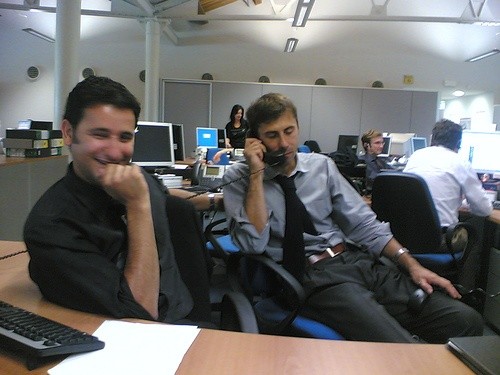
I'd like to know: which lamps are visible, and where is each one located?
[292,0,315,27]
[284,38,299,53]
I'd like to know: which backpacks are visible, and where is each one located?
[329,152,357,183]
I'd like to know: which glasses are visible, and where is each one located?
[371,141,386,145]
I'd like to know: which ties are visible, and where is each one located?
[272,171,322,312]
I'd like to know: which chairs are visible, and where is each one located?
[165,141,478,340]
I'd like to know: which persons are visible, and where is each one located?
[225,105,248,148]
[357,129,395,196]
[403,119,500,284]
[24,75,222,328]
[222,93,484,342]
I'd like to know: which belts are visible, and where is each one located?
[307,241,354,266]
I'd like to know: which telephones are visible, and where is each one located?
[230,148,247,163]
[192,162,227,191]
[245,129,286,167]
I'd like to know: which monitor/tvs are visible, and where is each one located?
[458,130,500,174]
[128,121,185,167]
[195,127,227,149]
[337,135,359,155]
[376,132,426,158]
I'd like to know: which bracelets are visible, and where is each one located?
[392,247,409,262]
[208,193,214,211]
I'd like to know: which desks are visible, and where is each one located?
[0,240,27,260]
[461,204,500,337]
[0,251,476,375]
[0,153,68,241]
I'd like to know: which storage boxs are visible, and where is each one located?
[0,121,64,158]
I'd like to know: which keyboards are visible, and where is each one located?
[0,301,105,371]
